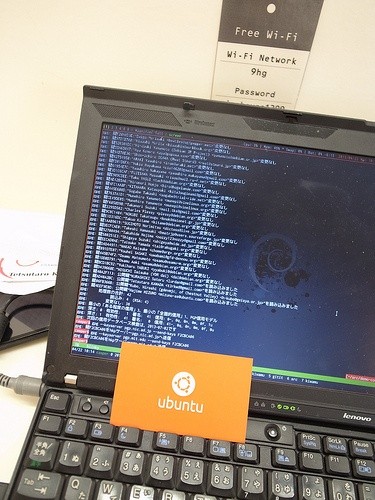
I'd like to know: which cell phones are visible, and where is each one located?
[1,285,56,352]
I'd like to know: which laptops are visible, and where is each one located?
[3,84,375,500]
